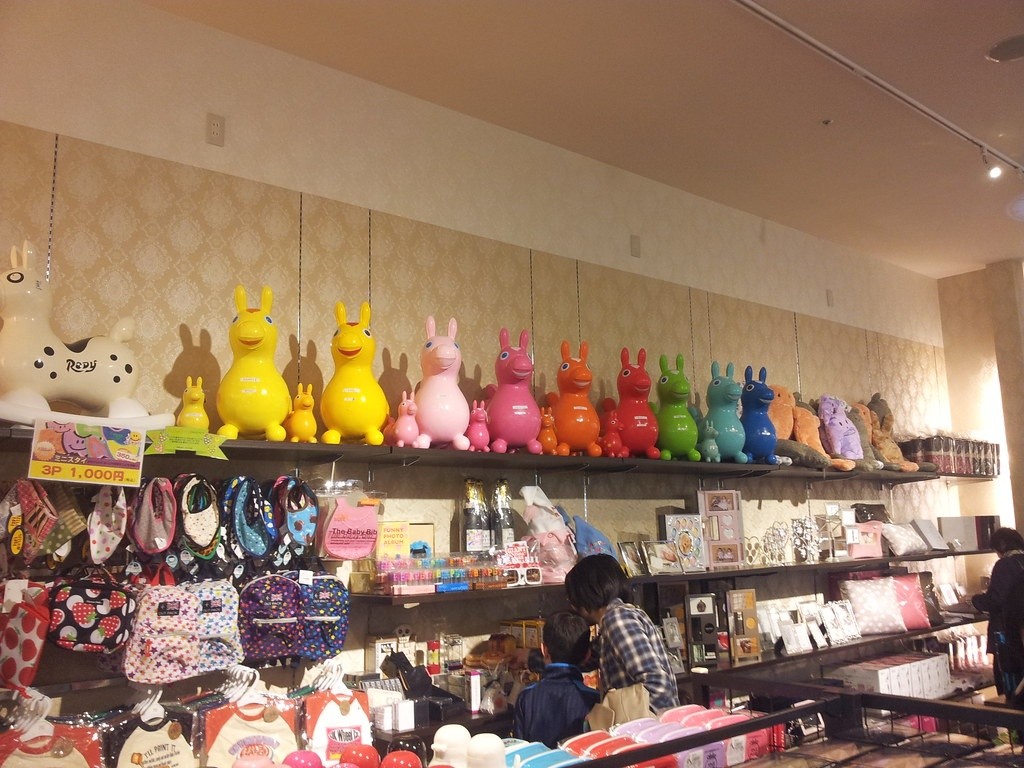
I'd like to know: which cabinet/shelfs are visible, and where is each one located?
[0,426,997,606]
[672,614,998,722]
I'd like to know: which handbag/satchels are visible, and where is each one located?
[0,579,50,691]
[49,552,352,686]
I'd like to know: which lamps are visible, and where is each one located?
[980,146,1003,179]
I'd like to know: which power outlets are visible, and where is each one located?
[205,112,226,148]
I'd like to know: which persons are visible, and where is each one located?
[504,611,601,750]
[972,527,1024,745]
[506,552,680,731]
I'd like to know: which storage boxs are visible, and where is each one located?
[365,636,399,680]
[910,519,950,552]
[821,651,984,732]
[937,516,966,552]
[829,566,909,600]
[464,671,480,714]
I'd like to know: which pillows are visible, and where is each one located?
[841,577,908,635]
[873,572,934,631]
[882,522,927,555]
[919,571,946,626]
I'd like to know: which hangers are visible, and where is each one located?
[309,658,353,699]
[6,686,53,741]
[127,677,166,721]
[218,664,267,708]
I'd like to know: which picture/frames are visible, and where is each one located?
[617,540,646,577]
[662,617,685,649]
[818,604,849,645]
[666,647,686,674]
[640,540,685,575]
[795,600,821,636]
[829,599,862,638]
